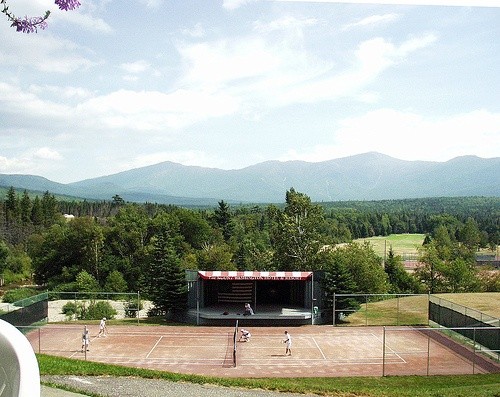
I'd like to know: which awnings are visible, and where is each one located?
[196,270,314,325]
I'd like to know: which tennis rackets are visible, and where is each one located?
[105,327,110,334]
[279,340,286,344]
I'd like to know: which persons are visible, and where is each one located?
[240,329,251,342]
[283,330,293,356]
[245,303,251,311]
[96,317,109,337]
[81,328,89,352]
